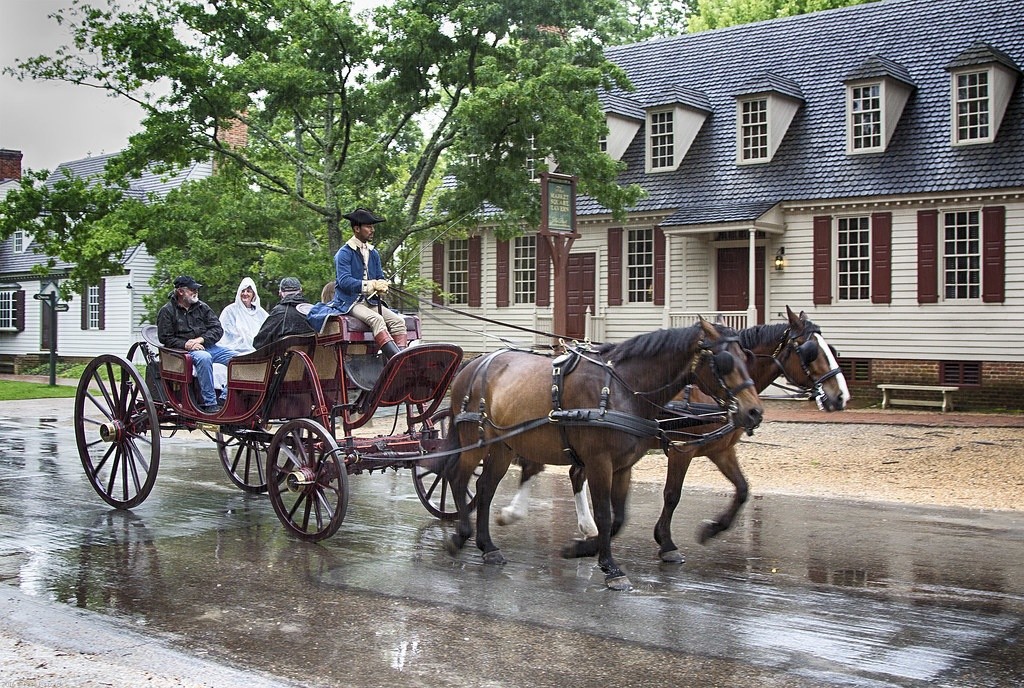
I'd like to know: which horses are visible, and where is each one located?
[415,305,850,591]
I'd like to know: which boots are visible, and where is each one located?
[380,339,401,359]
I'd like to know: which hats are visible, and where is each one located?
[342,208,386,223]
[280,277,301,291]
[173,275,203,289]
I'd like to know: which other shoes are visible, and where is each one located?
[205,405,220,412]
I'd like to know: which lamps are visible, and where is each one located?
[775,247,785,270]
[126,282,133,290]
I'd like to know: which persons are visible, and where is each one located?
[156,276,240,413]
[252,278,315,351]
[307,209,412,361]
[215,277,270,391]
[321,282,338,305]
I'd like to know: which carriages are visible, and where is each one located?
[73,280,851,592]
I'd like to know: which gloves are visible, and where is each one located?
[370,279,392,296]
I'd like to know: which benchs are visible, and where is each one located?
[877,383,960,413]
[316,282,422,347]
[142,324,360,391]
